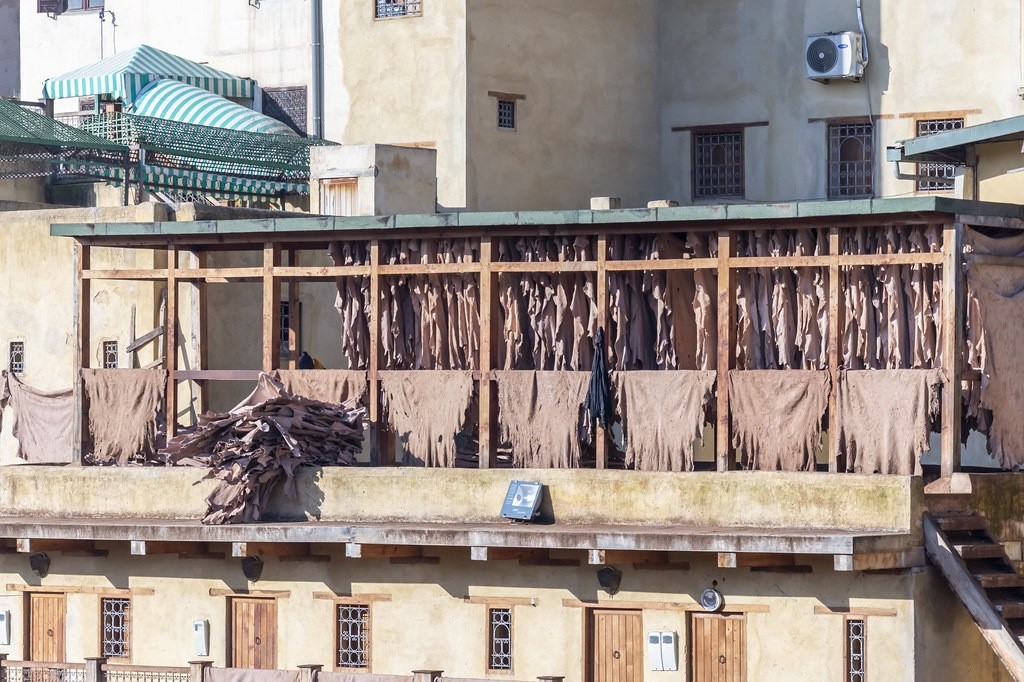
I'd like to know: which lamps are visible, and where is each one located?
[500,480,544,526]
[701,588,722,611]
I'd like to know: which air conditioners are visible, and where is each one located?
[804,31,864,81]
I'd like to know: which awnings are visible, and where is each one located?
[140,165,310,203]
[51,157,139,187]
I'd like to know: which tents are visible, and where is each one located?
[38,44,254,185]
[128,79,309,179]
